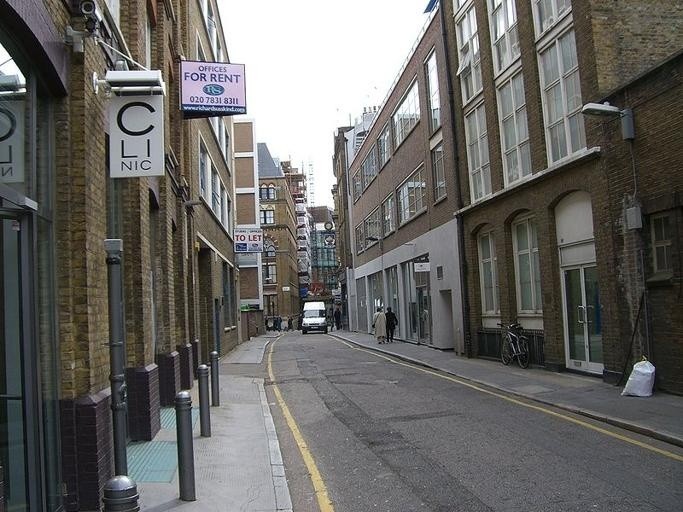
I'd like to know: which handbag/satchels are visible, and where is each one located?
[371,322,375,327]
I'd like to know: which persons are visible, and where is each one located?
[386,306,398,344]
[334,308,342,329]
[373,308,390,345]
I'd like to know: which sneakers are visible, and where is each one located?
[378,339,393,343]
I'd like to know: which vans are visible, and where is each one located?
[301,301,328,334]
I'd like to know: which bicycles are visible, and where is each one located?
[497,323,530,369]
